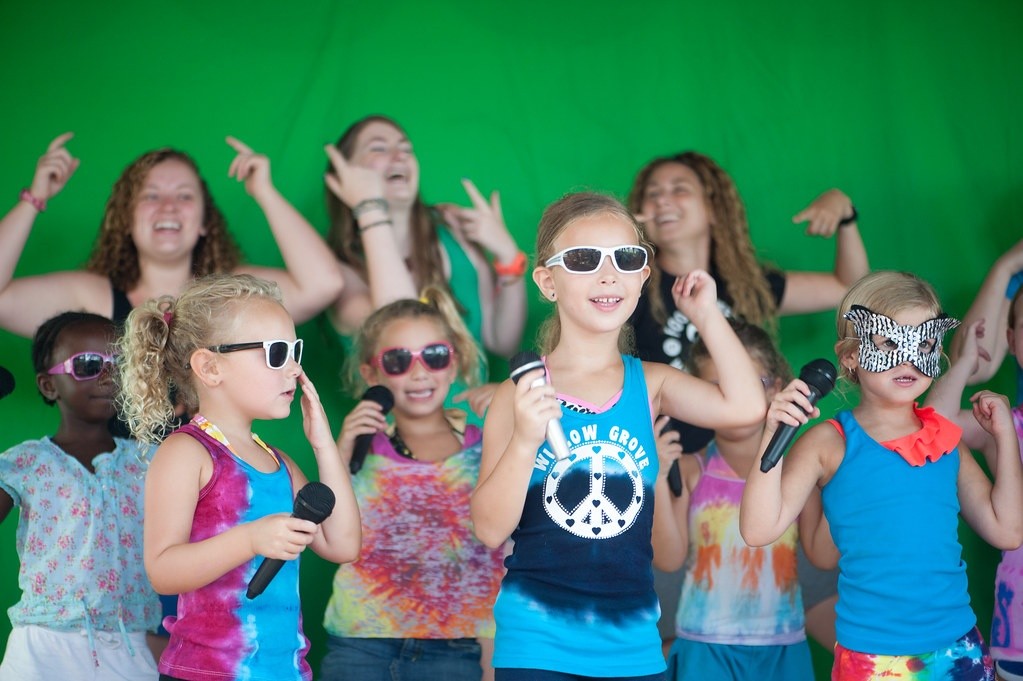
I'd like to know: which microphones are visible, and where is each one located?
[760,358,837,473]
[656,415,683,496]
[510,350,571,461]
[349,385,394,476]
[246,482,336,600]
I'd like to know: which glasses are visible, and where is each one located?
[371,341,456,378]
[47,351,121,381]
[545,244,649,274]
[185,338,303,371]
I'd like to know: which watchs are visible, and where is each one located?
[840,207,857,224]
[494,253,527,276]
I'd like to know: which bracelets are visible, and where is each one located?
[360,219,394,237]
[354,198,388,215]
[500,279,520,285]
[20,188,45,212]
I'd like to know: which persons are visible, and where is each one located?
[628,151,870,669]
[316,285,500,681]
[0,311,165,681]
[0,131,346,656]
[470,191,767,681]
[738,273,1023,681]
[650,313,839,681]
[950,240,1023,386]
[109,271,363,681]
[923,283,1023,681]
[323,113,525,430]
